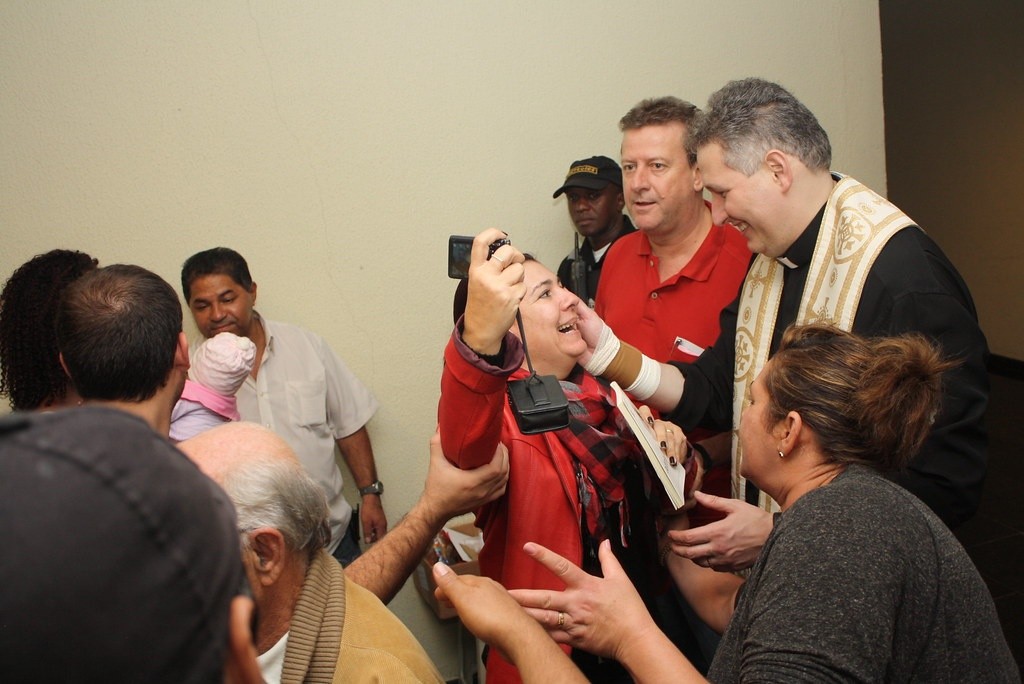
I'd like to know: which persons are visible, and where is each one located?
[0,246,511,683]
[438,75,1022,684]
[510,322,1024,684]
[174,420,447,683]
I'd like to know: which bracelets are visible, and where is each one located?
[692,443,713,474]
[660,541,673,566]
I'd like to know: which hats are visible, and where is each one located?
[187,331,257,398]
[553,155,621,199]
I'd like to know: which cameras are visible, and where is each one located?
[447,234,512,280]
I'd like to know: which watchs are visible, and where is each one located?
[359,481,384,495]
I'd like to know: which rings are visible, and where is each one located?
[667,429,674,435]
[558,613,564,625]
[491,253,505,270]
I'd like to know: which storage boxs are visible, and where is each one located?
[413,523,482,620]
